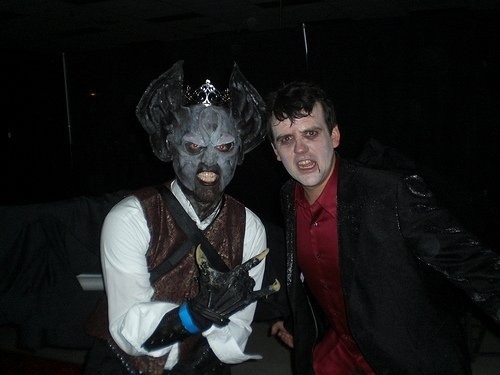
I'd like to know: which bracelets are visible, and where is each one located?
[179,301,201,336]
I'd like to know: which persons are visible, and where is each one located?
[257,81,500,375]
[84,45,281,375]
